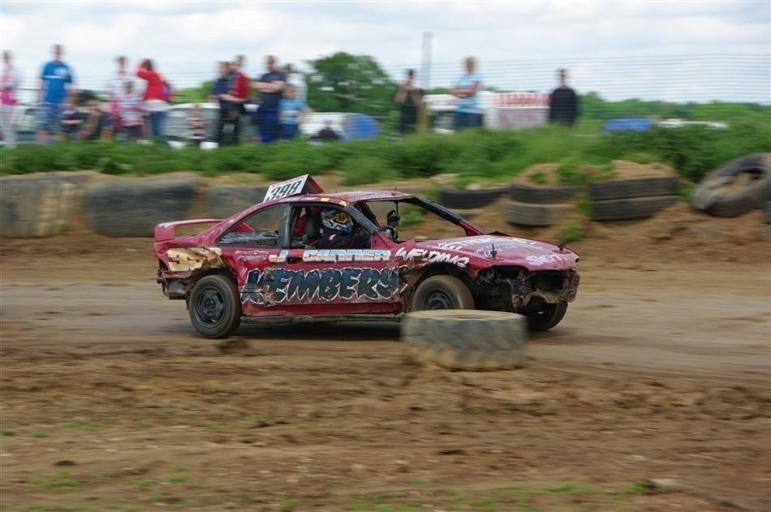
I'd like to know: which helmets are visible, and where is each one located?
[320,207,354,234]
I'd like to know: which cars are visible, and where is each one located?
[152,174,582,339]
[154,103,264,149]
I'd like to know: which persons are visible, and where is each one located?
[318,207,398,249]
[448,54,487,133]
[395,69,425,134]
[184,104,211,146]
[207,53,306,147]
[0,49,21,146]
[34,45,168,147]
[548,69,578,126]
